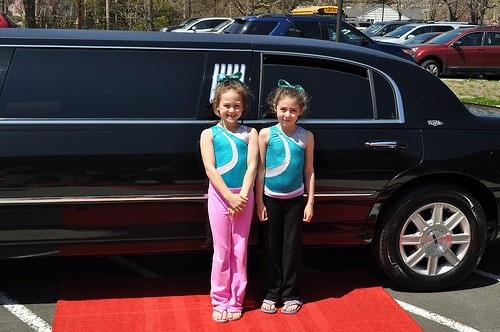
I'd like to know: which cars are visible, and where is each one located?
[220,5,417,63]
[345,17,480,45]
[405,27,500,79]
[0,27,500,294]
[160,17,237,34]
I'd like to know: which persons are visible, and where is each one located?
[199,72,260,324]
[256,79,315,316]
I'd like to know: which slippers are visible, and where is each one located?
[281,299,303,314]
[212,305,243,323]
[261,299,277,314]
